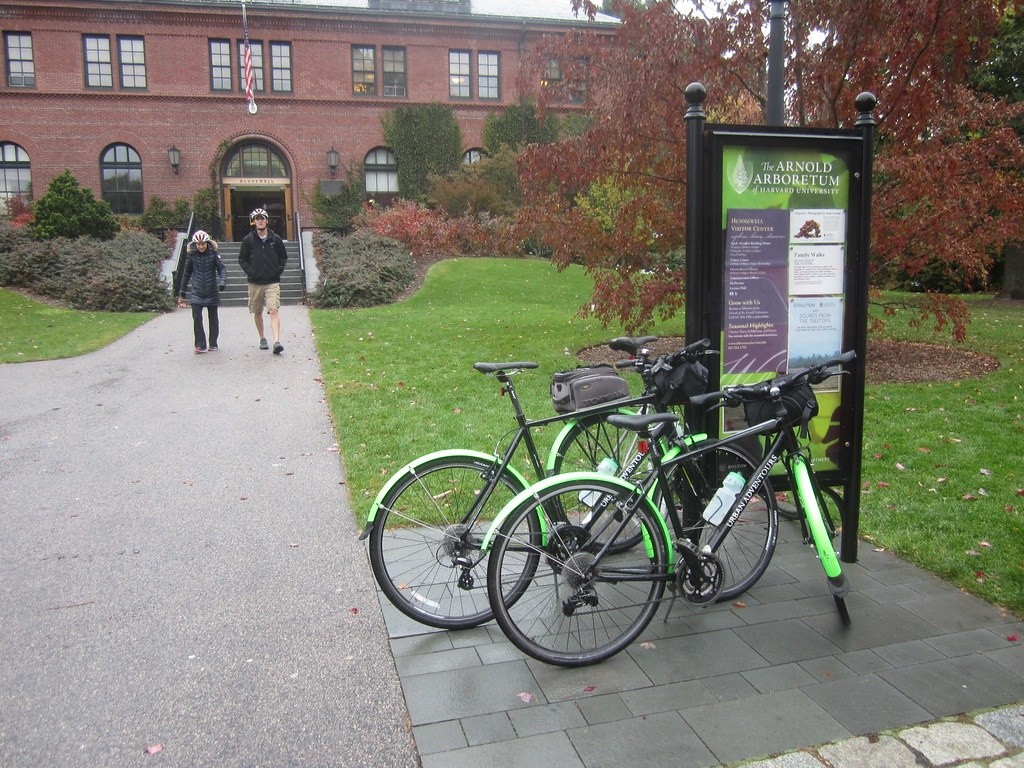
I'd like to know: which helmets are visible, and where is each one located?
[192,231,210,243]
[251,208,269,220]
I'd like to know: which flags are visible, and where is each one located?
[242,4,255,101]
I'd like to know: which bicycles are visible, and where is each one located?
[480,349,861,670]
[356,332,781,633]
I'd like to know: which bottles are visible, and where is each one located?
[702,472,744,525]
[579,458,617,508]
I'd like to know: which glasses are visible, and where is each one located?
[254,215,266,220]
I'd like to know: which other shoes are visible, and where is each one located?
[260,341,268,349]
[272,343,283,354]
[209,346,217,351]
[195,347,202,353]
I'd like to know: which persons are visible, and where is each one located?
[180,230,226,353]
[238,208,287,355]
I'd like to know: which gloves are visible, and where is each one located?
[181,293,186,297]
[220,286,224,291]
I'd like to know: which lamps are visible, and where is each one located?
[168,141,181,176]
[326,145,339,176]
